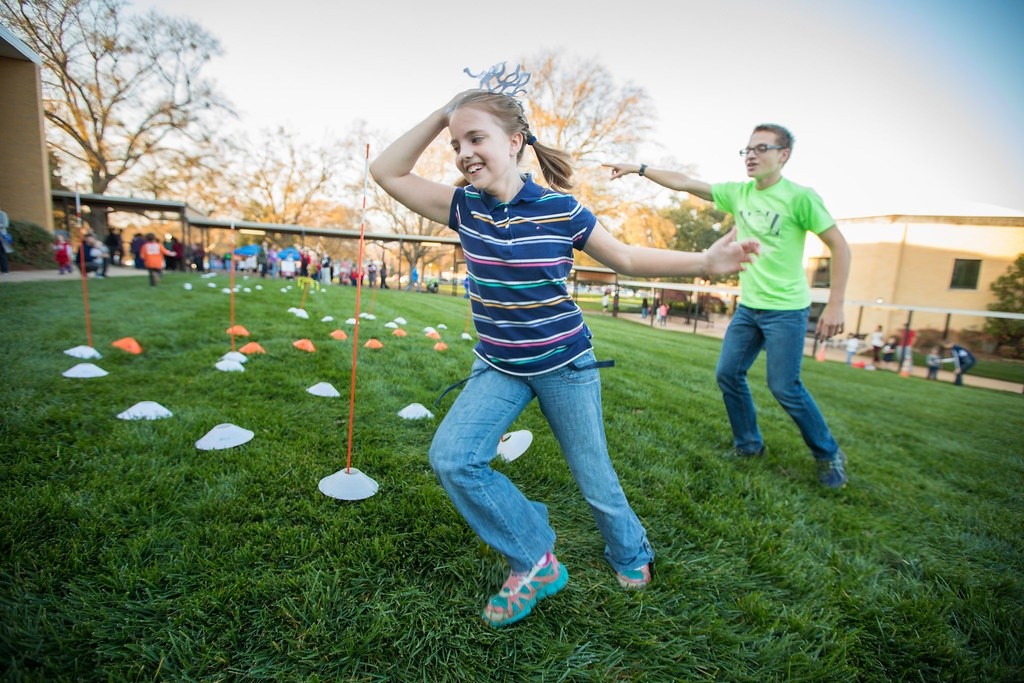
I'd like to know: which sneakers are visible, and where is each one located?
[617,564,653,588]
[819,450,850,490]
[482,551,569,627]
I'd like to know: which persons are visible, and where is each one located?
[338,257,389,289]
[49,222,205,281]
[655,299,670,328]
[0,210,15,273]
[463,271,470,298]
[404,263,421,292]
[256,240,334,282]
[868,320,918,377]
[816,340,828,361]
[602,292,610,313]
[368,87,760,628]
[601,124,851,491]
[926,345,942,380]
[642,299,647,318]
[140,233,177,288]
[843,332,859,365]
[939,339,975,386]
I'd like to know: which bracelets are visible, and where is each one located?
[638,164,648,176]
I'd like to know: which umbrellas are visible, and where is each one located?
[234,243,262,256]
[277,246,300,260]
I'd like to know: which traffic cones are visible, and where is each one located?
[898,350,915,379]
[816,340,830,363]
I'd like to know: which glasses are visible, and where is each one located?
[739,143,782,157]
